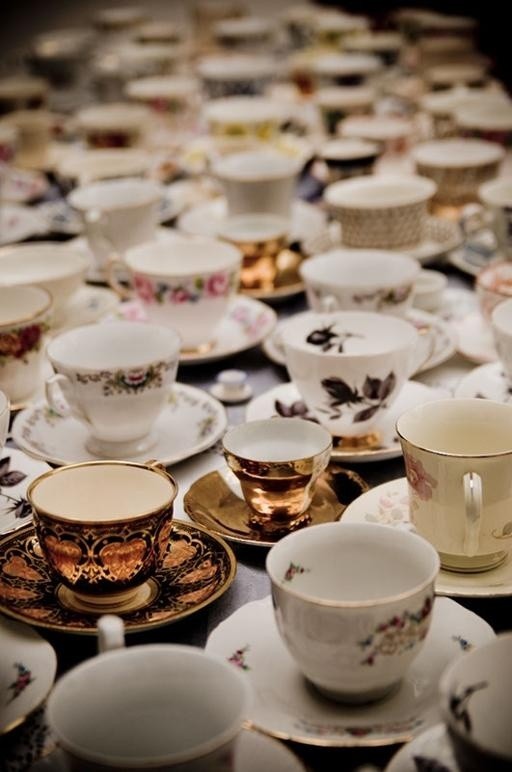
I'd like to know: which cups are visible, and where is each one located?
[274,311,423,444]
[0,393,12,461]
[439,632,512,771]
[391,400,511,576]
[0,243,92,303]
[2,2,510,149]
[301,249,418,310]
[477,264,512,312]
[111,241,241,335]
[70,179,161,242]
[29,461,185,597]
[415,139,500,196]
[324,174,435,248]
[211,152,300,215]
[480,178,510,264]
[49,322,183,457]
[265,517,446,704]
[219,419,337,532]
[72,229,173,279]
[492,297,511,373]
[44,614,251,771]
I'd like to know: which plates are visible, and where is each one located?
[203,587,498,749]
[452,303,510,357]
[325,216,462,256]
[10,379,228,466]
[183,462,374,548]
[244,378,442,461]
[2,446,52,541]
[379,721,474,771]
[183,198,324,244]
[114,292,274,364]
[38,714,304,771]
[340,475,511,604]
[0,613,60,742]
[2,517,241,637]
[271,310,453,374]
